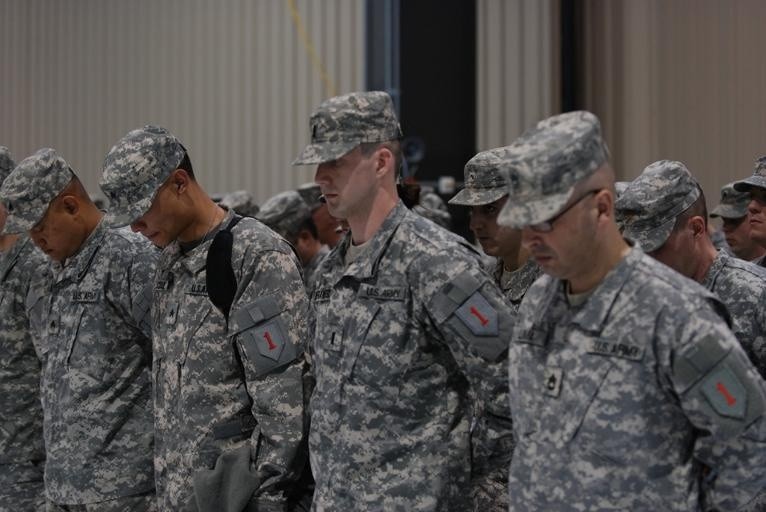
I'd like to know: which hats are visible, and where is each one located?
[0,148,76,237]
[448,146,510,205]
[496,110,609,229]
[710,183,750,218]
[733,156,766,192]
[221,191,253,218]
[298,184,322,210]
[614,160,703,253]
[256,192,313,239]
[291,91,404,166]
[99,124,187,229]
[0,146,16,187]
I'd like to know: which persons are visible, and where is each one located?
[734,156,765,268]
[709,179,766,266]
[0,145,45,511]
[97,126,309,512]
[214,178,349,290]
[495,111,766,512]
[0,148,162,512]
[291,90,518,512]
[447,145,543,307]
[613,160,765,376]
